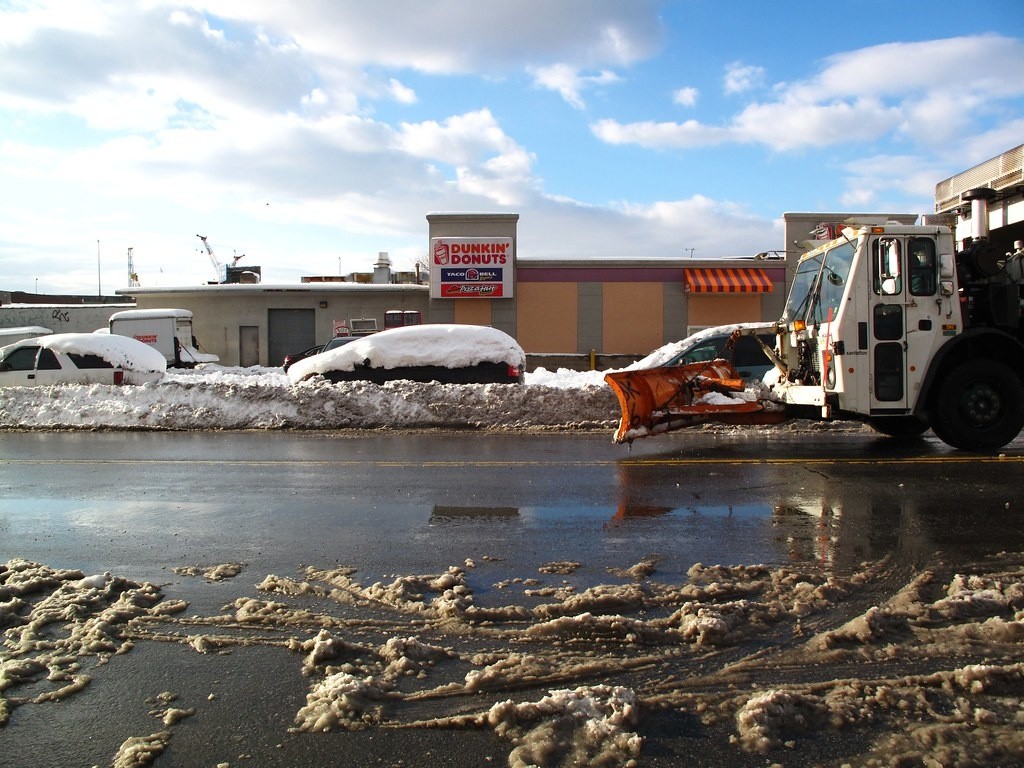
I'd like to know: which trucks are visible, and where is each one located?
[0,325,52,350]
[93,308,220,369]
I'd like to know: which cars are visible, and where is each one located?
[288,323,528,388]
[282,344,328,375]
[0,334,167,388]
[319,335,367,356]
[657,322,778,384]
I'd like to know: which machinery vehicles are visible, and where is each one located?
[604,142,1024,455]
